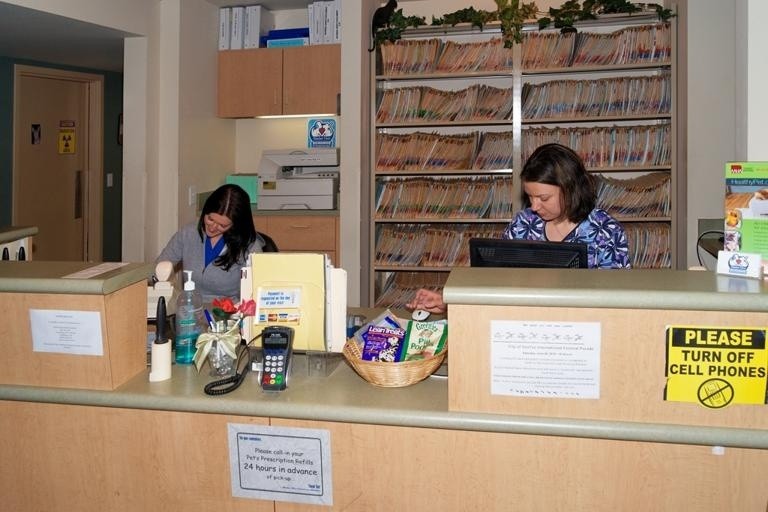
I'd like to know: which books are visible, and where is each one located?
[520,22,670,268]
[375,39,517,308]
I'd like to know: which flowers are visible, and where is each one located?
[212,296,256,333]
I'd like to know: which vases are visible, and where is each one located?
[208,327,238,375]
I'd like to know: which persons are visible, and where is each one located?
[147,184,266,313]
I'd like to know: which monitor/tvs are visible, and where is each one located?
[468,237,589,270]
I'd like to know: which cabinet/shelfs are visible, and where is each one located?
[216,43,341,117]
[370,13,676,317]
[253,214,340,271]
[697,218,725,271]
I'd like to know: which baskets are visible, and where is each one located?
[342,332,451,388]
